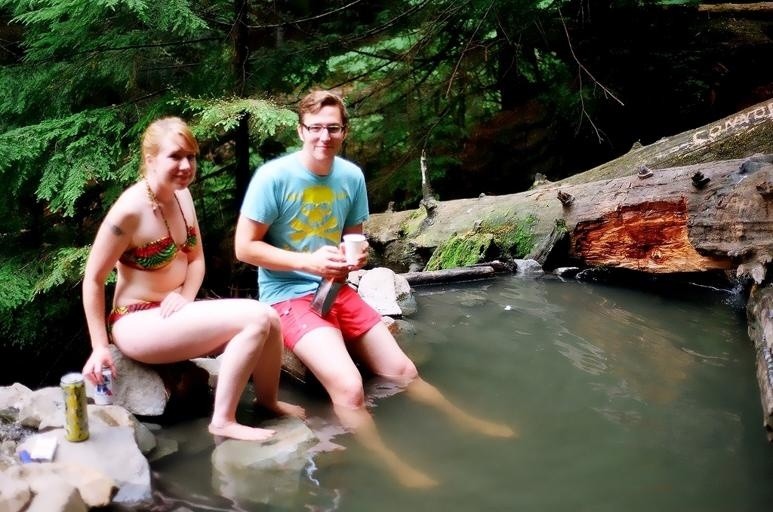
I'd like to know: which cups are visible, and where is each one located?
[343,233,366,266]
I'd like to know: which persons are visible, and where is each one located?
[231,87,522,494]
[79,114,306,445]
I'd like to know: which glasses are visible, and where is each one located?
[301,123,345,133]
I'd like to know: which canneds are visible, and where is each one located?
[58,373,90,442]
[93,367,113,406]
